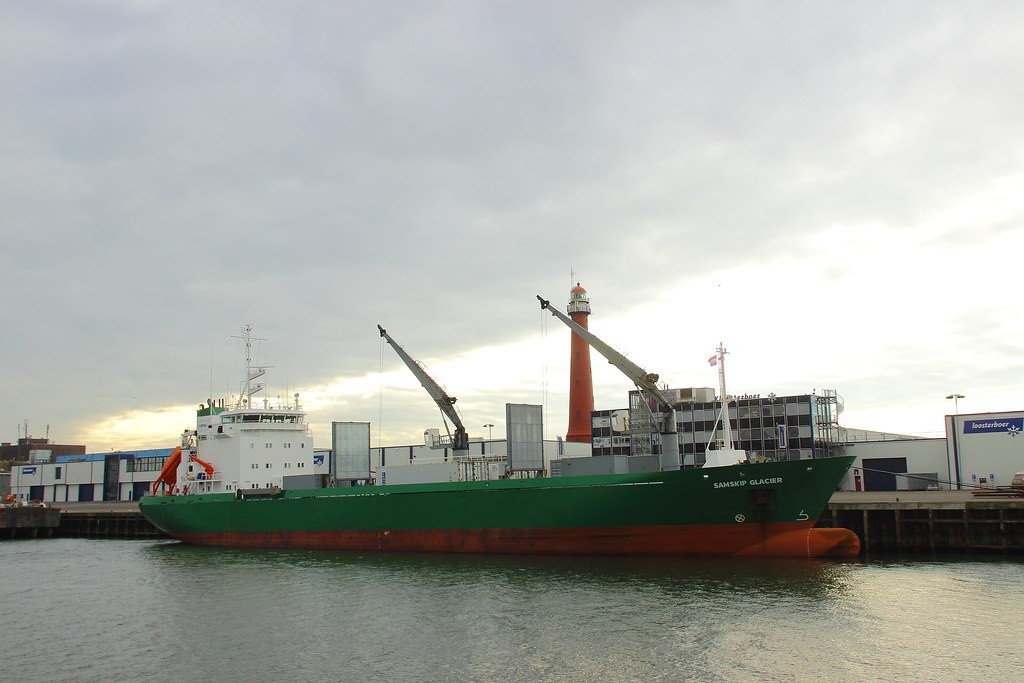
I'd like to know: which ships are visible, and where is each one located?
[137,294,863,562]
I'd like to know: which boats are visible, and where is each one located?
[151,447,182,499]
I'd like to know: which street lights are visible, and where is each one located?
[946,394,965,491]
[482,424,495,455]
